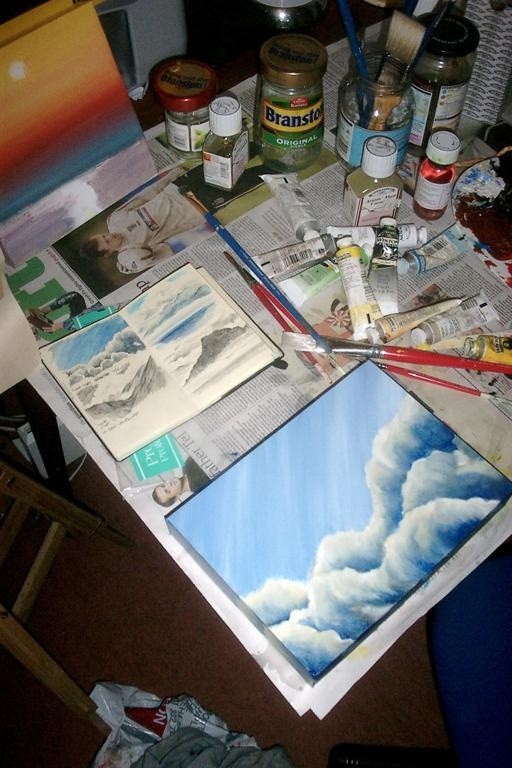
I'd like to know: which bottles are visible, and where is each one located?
[413,130,461,222]
[163,109,210,161]
[201,98,253,191]
[343,138,402,228]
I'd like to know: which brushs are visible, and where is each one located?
[367,9,427,130]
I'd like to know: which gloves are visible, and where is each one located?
[115,262,130,275]
[170,167,190,182]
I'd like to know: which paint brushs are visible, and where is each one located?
[243,268,346,377]
[177,185,339,361]
[375,362,512,405]
[224,251,332,385]
[279,331,512,375]
[407,1,453,75]
[339,0,374,110]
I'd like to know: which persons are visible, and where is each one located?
[80,138,284,274]
[152,455,212,507]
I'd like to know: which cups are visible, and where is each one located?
[337,57,413,166]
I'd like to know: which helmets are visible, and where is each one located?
[117,241,155,272]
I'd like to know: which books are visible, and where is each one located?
[38,262,283,461]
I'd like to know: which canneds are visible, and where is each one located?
[148,54,217,158]
